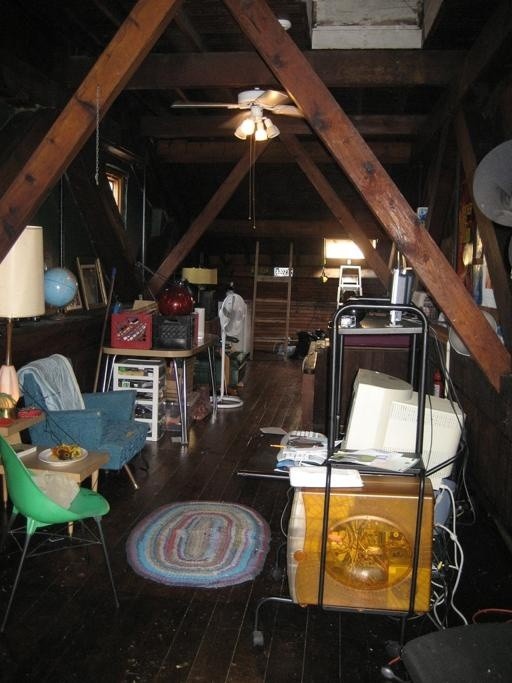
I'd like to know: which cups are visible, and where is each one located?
[340,316,355,330]
[195,308,206,339]
[198,339,204,346]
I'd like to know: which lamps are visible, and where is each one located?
[0,224,47,419]
[181,268,217,305]
[235,104,280,142]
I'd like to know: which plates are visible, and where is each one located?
[39,446,90,466]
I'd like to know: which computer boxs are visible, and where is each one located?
[283,475,435,613]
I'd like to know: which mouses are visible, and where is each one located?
[276,459,295,471]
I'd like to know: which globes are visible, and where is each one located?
[45,267,78,320]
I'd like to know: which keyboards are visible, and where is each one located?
[276,430,328,465]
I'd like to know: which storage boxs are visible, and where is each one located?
[163,391,200,432]
[166,356,197,398]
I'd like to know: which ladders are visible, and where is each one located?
[336,266,363,312]
[249,240,292,363]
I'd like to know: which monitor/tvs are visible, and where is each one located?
[342,368,467,490]
[384,267,407,328]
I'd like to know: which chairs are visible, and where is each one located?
[17,352,148,490]
[0,433,120,634]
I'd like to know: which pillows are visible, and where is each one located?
[31,474,80,509]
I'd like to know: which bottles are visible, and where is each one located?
[343,303,366,323]
[433,368,444,397]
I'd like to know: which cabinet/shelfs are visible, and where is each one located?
[113,358,167,442]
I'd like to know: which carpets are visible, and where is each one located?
[125,500,273,589]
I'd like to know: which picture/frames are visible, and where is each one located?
[64,288,84,313]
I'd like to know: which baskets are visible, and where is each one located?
[110,311,153,351]
[150,309,196,349]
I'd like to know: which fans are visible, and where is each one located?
[209,293,249,409]
[170,86,305,130]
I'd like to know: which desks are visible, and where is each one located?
[101,332,220,445]
[0,446,111,534]
